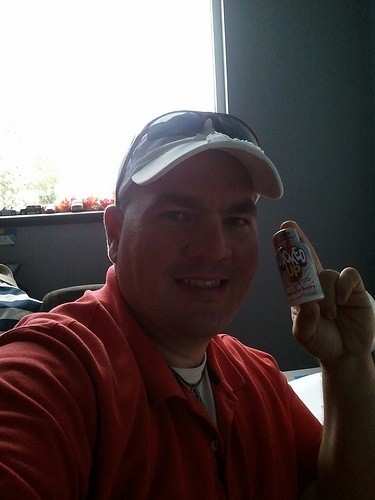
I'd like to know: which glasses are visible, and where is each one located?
[116,112,262,193]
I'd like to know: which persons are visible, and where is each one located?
[0,111,374,500]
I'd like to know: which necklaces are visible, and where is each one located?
[165,355,208,410]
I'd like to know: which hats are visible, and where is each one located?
[115,109,284,200]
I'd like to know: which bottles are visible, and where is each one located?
[272,228,325,306]
